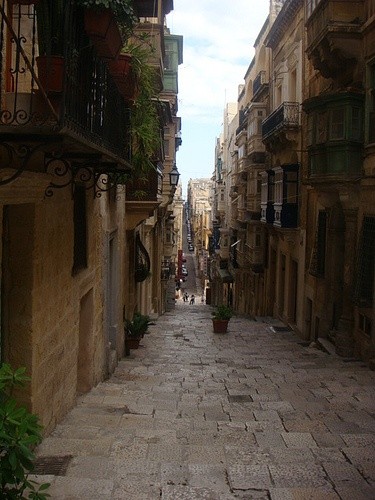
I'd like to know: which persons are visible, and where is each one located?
[183,292,195,306]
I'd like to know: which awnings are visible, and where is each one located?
[218,269,232,284]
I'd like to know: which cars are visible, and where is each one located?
[180,265,188,276]
[187,219,194,252]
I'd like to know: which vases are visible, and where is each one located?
[4,92,34,128]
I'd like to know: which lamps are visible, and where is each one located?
[168,163,180,186]
[134,230,150,283]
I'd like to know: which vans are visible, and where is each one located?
[182,253,188,262]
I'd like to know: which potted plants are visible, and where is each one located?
[212,304,233,333]
[125,312,152,349]
[36,0,165,201]
